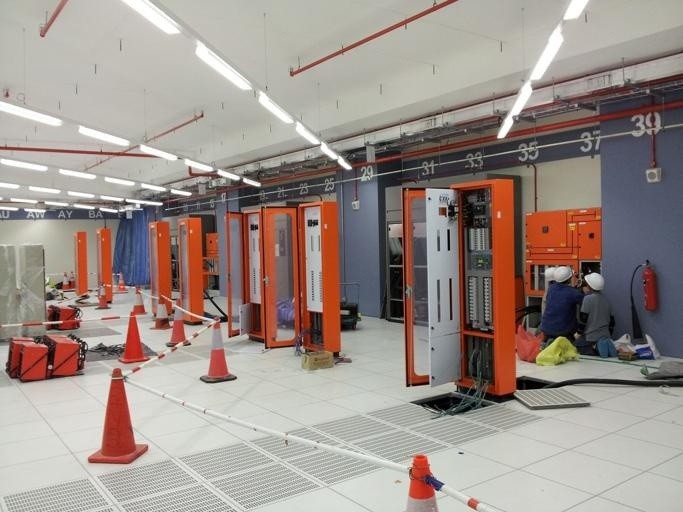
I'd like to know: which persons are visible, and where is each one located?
[571,272,618,358]
[539,266,556,318]
[537,265,587,347]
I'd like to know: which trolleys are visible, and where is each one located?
[340,283,362,330]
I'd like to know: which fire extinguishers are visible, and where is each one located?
[630,259,657,339]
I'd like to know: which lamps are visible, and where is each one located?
[140,145,261,188]
[1,159,48,172]
[104,177,135,187]
[495,1,592,140]
[295,122,353,172]
[59,169,97,181]
[1,183,192,214]
[193,39,252,93]
[259,93,295,127]
[76,125,131,148]
[2,101,63,129]
[127,1,182,36]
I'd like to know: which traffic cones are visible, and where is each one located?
[118,310,151,363]
[404,454,442,512]
[129,284,149,315]
[200,317,239,384]
[150,295,173,331]
[167,298,194,347]
[61,270,129,310]
[86,368,149,466]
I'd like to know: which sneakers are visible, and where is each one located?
[595,336,618,359]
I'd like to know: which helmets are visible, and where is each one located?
[553,265,573,283]
[584,271,605,292]
[544,266,556,282]
